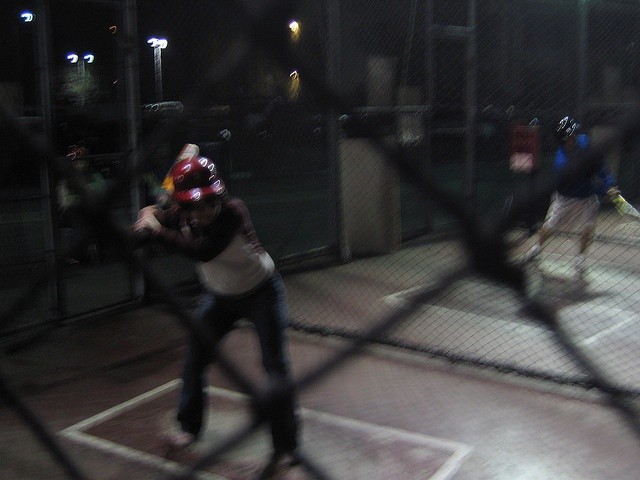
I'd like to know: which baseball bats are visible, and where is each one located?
[137,142,200,236]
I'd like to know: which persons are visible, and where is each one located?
[54,140,116,269]
[133,154,311,480]
[508,115,621,287]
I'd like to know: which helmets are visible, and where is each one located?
[171,157,228,204]
[556,115,581,140]
